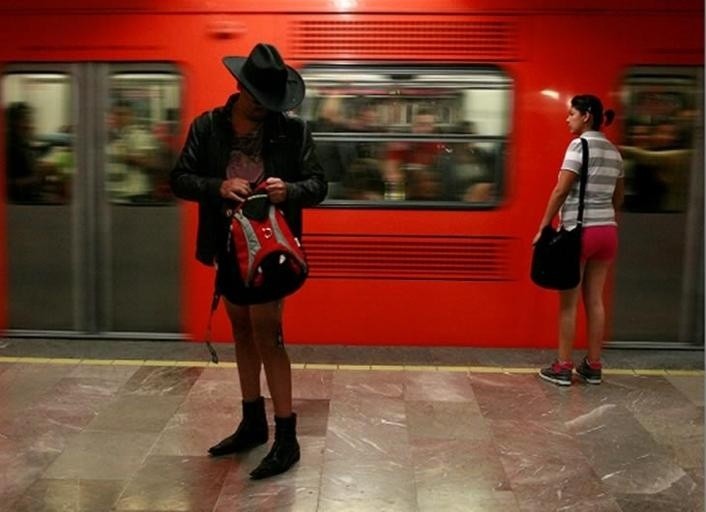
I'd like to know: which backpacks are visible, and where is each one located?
[205,182,310,364]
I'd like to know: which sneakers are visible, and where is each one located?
[575,356,602,384]
[538,359,573,386]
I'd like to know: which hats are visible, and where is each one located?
[222,44,305,112]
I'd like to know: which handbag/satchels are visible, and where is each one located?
[530,222,581,291]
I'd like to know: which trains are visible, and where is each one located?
[0,2,706,347]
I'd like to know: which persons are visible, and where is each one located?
[2,99,180,205]
[616,90,698,217]
[167,43,329,478]
[305,89,496,203]
[530,95,625,387]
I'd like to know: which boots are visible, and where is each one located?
[207,395,268,456]
[249,412,301,479]
